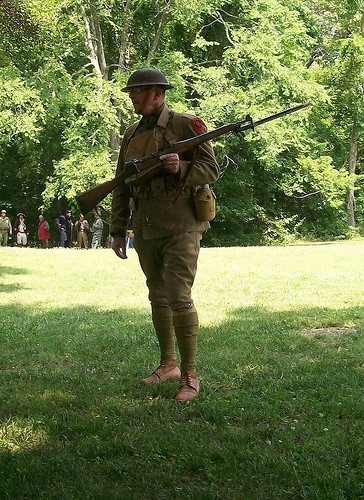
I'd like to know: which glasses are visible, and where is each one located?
[125,85,154,94]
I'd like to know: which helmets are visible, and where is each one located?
[119,69,170,92]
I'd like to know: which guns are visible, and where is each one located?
[74,100,312,218]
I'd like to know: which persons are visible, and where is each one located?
[75,213,90,249]
[54,210,73,247]
[0,209,12,247]
[37,215,50,248]
[91,210,103,249]
[109,69,221,402]
[15,213,29,247]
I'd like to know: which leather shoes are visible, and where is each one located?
[174,373,199,401]
[140,359,181,384]
